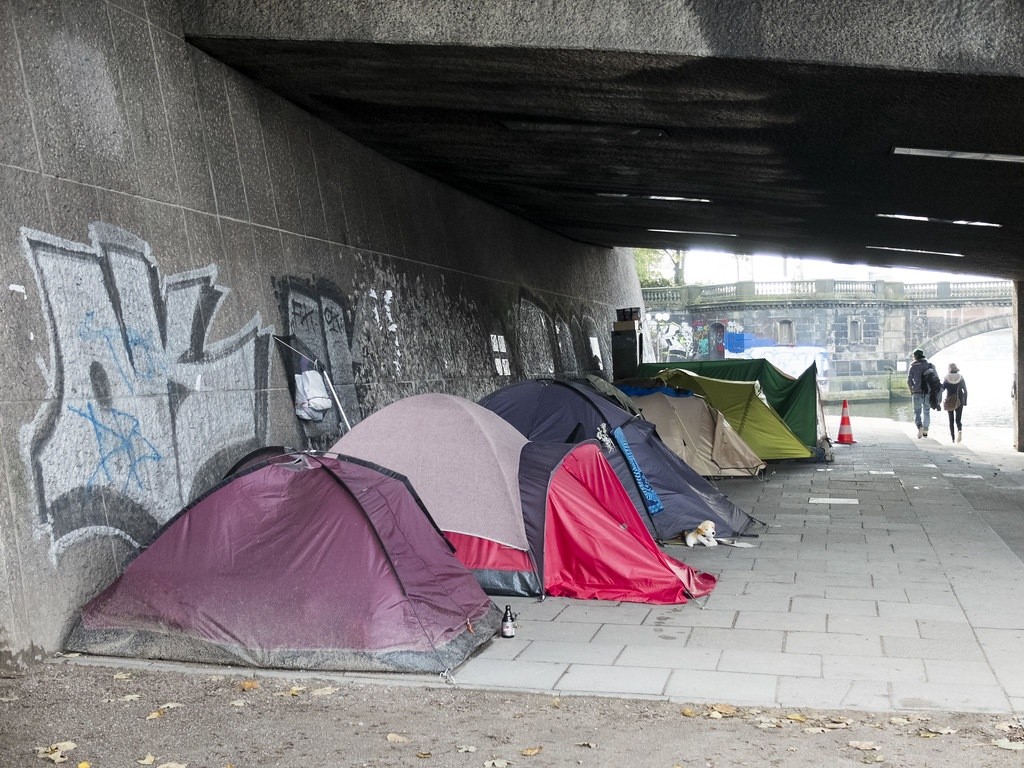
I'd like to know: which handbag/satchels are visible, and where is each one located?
[944,391,961,411]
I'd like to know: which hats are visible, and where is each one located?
[948,364,959,372]
[914,350,923,356]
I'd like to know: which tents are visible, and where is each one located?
[475,377,768,539]
[323,392,716,608]
[65,447,511,676]
[572,357,836,492]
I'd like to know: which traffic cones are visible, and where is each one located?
[833,399,857,444]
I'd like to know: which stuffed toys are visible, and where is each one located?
[684,519,718,548]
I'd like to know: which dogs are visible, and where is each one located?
[684,520,718,547]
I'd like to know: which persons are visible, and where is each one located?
[942,363,968,443]
[908,349,936,439]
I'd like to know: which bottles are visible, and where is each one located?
[502,604,516,638]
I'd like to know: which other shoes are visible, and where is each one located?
[923,432,927,438]
[957,430,962,443]
[918,427,924,439]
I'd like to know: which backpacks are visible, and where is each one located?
[922,363,940,394]
